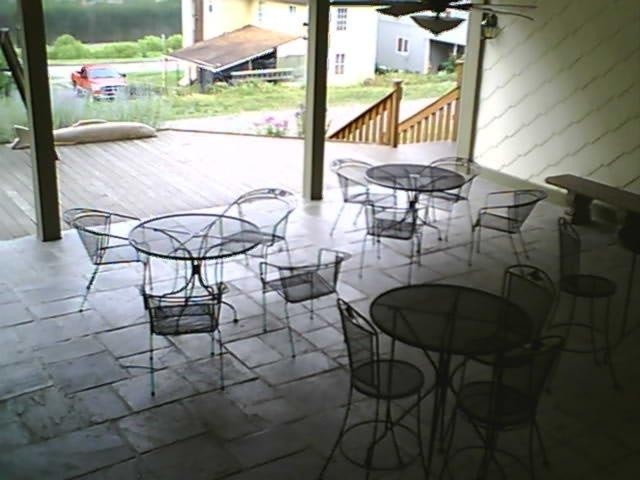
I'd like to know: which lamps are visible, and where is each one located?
[479,13,501,38]
[410,11,465,35]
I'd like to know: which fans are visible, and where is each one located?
[330,0,538,21]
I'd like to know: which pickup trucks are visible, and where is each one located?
[71,64,131,100]
